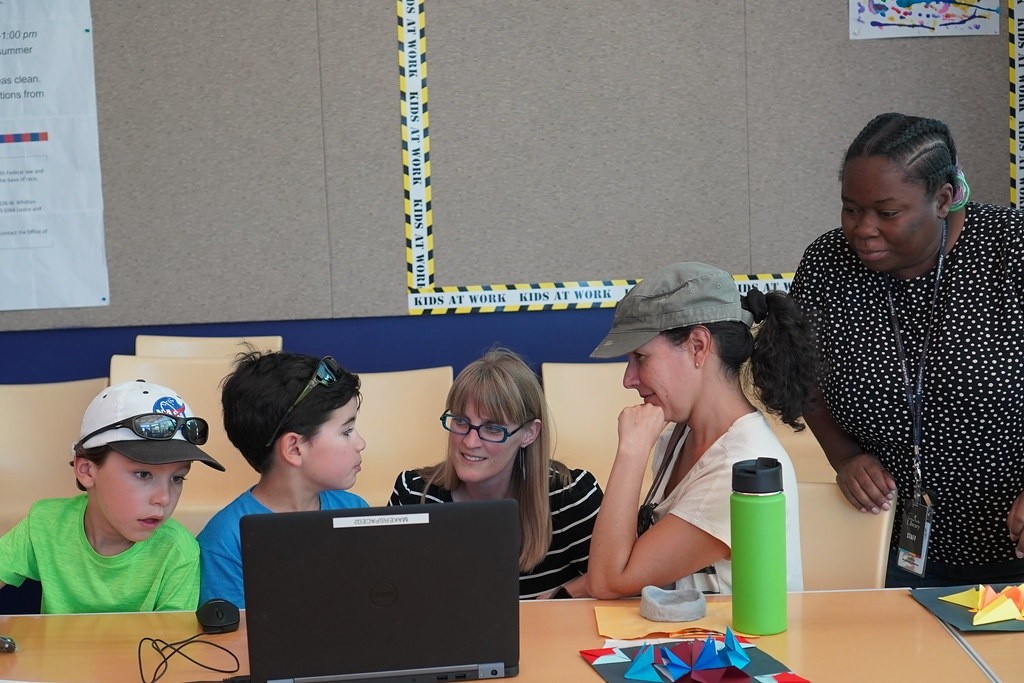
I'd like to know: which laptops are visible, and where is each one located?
[239,497,520,683]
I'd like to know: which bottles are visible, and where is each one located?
[729,456,789,637]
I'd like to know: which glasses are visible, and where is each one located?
[74,413,209,445]
[266,355,346,448]
[438,407,538,443]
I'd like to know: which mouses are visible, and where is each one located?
[196,598,241,635]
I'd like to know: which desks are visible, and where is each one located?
[0,584,1023,683]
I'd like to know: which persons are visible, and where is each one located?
[387,352,606,601]
[0,378,226,614]
[787,112,1024,590]
[585,262,816,600]
[196,353,370,609]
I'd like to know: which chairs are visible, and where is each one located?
[0,337,898,592]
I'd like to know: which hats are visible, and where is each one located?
[80,380,226,472]
[588,262,758,358]
